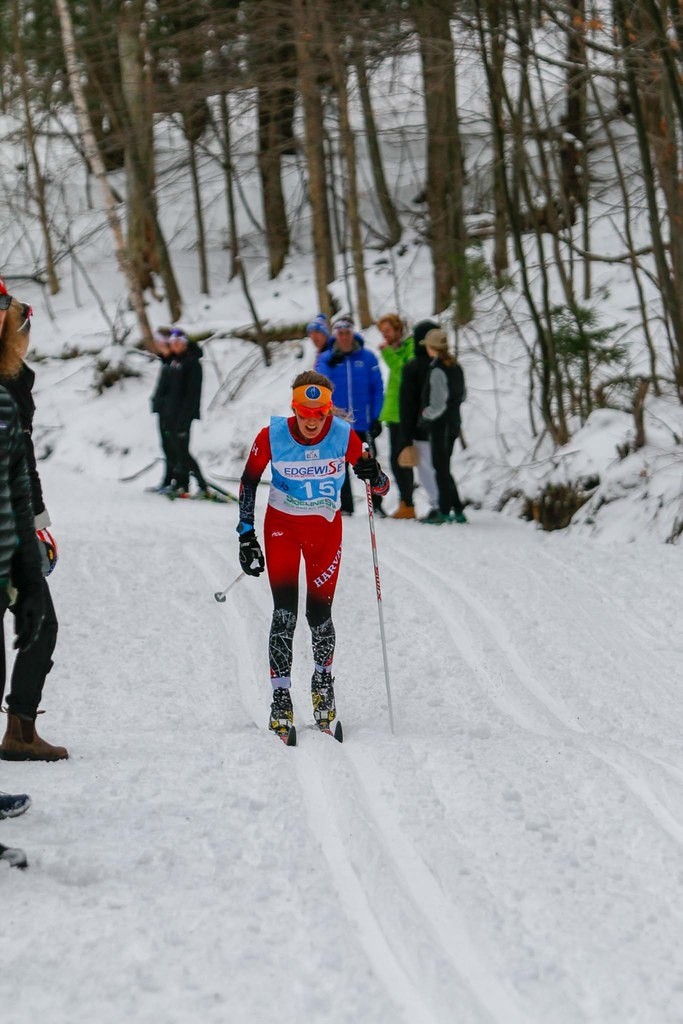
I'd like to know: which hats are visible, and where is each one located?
[420,329,448,347]
[306,314,330,336]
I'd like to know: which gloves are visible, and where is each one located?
[368,419,382,439]
[13,584,45,651]
[352,457,381,483]
[35,528,58,577]
[239,528,265,577]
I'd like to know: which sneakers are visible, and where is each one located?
[311,668,336,722]
[269,687,294,730]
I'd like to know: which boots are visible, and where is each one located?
[0,706,69,761]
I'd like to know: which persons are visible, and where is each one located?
[236,371,390,732]
[377,315,468,526]
[309,313,386,518]
[148,326,211,500]
[0,279,70,868]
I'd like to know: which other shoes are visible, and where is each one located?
[0,844,27,867]
[340,507,351,516]
[420,510,467,524]
[391,501,415,518]
[374,507,387,518]
[0,793,31,818]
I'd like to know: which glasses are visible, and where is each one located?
[0,294,12,310]
[293,401,331,419]
[16,302,33,332]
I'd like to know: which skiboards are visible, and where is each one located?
[266,721,343,746]
[145,485,230,503]
